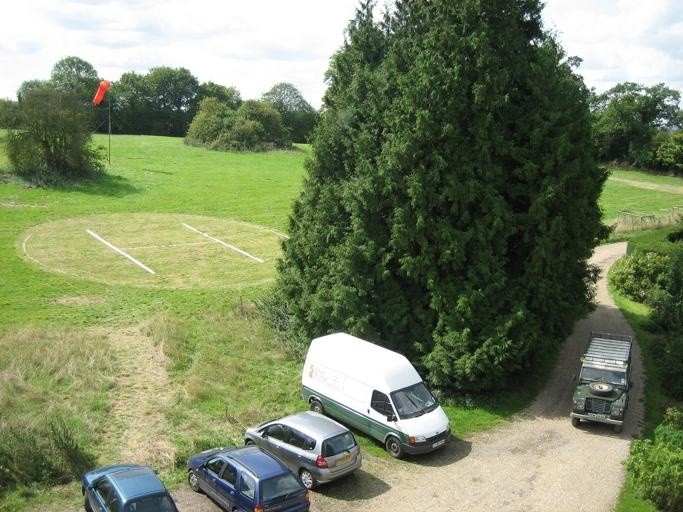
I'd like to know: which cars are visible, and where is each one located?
[245,410,362,489]
[81,463,178,511]
[187,445,310,512]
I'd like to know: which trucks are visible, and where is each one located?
[569,331,634,434]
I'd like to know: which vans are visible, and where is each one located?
[301,332,451,459]
